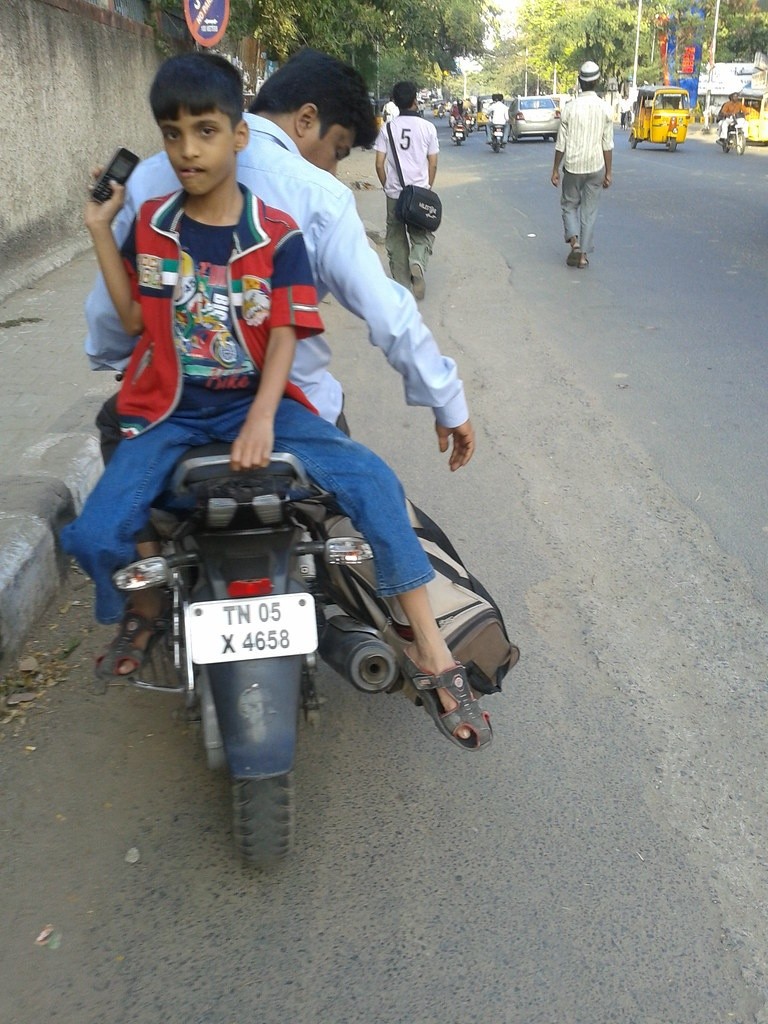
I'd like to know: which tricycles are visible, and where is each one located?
[476,95,496,131]
[433,101,446,119]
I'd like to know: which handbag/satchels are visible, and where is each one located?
[297,478,521,702]
[395,184,442,232]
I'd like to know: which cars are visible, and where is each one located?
[508,94,562,143]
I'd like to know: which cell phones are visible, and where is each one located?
[91,146,140,204]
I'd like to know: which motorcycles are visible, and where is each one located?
[447,110,476,147]
[627,84,689,152]
[438,112,444,118]
[716,112,749,155]
[486,115,504,153]
[95,367,398,874]
[738,89,768,144]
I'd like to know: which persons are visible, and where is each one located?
[715,92,750,144]
[57,48,494,752]
[550,61,615,269]
[372,81,440,301]
[381,93,509,148]
[620,95,632,129]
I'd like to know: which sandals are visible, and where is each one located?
[399,646,494,753]
[578,252,589,268]
[95,587,170,682]
[566,247,582,267]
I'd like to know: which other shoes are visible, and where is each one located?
[410,263,425,300]
[720,138,726,143]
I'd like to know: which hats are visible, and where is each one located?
[579,61,600,82]
